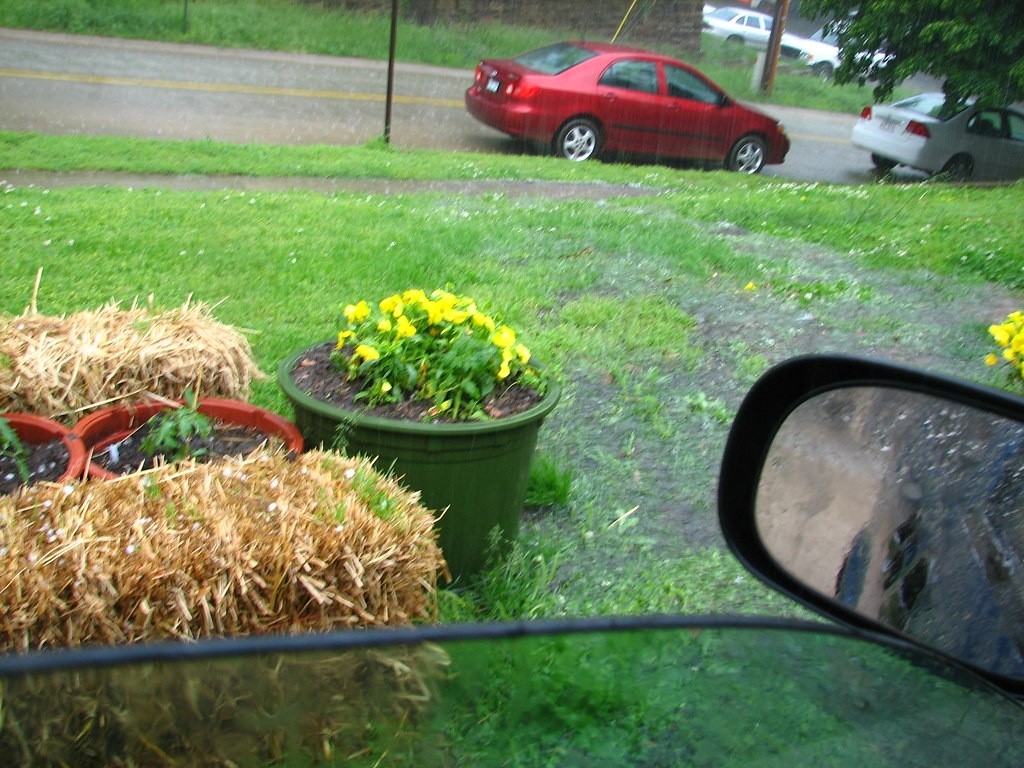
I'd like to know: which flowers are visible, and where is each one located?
[335,285,544,423]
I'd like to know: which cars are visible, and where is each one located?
[849,90,1024,189]
[770,10,898,90]
[696,5,787,57]
[464,37,790,181]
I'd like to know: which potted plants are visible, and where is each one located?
[278,326,561,586]
[0,388,299,506]
